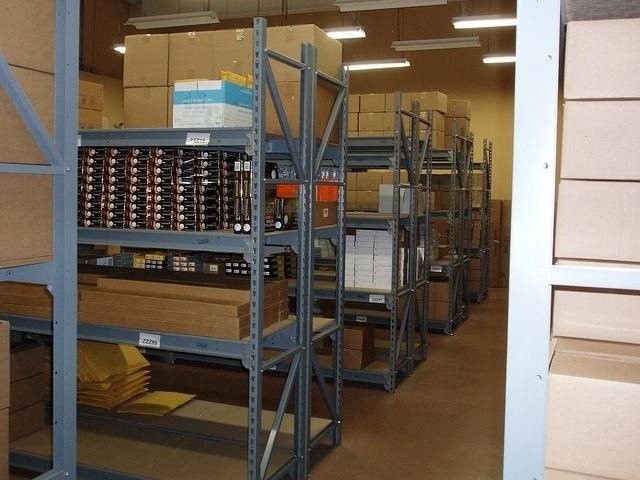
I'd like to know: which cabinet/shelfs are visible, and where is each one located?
[500,0,640,480]
[0,0,77,480]
[156,91,493,395]
[1,16,348,479]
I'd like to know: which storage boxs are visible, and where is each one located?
[78,24,343,141]
[543,16,640,479]
